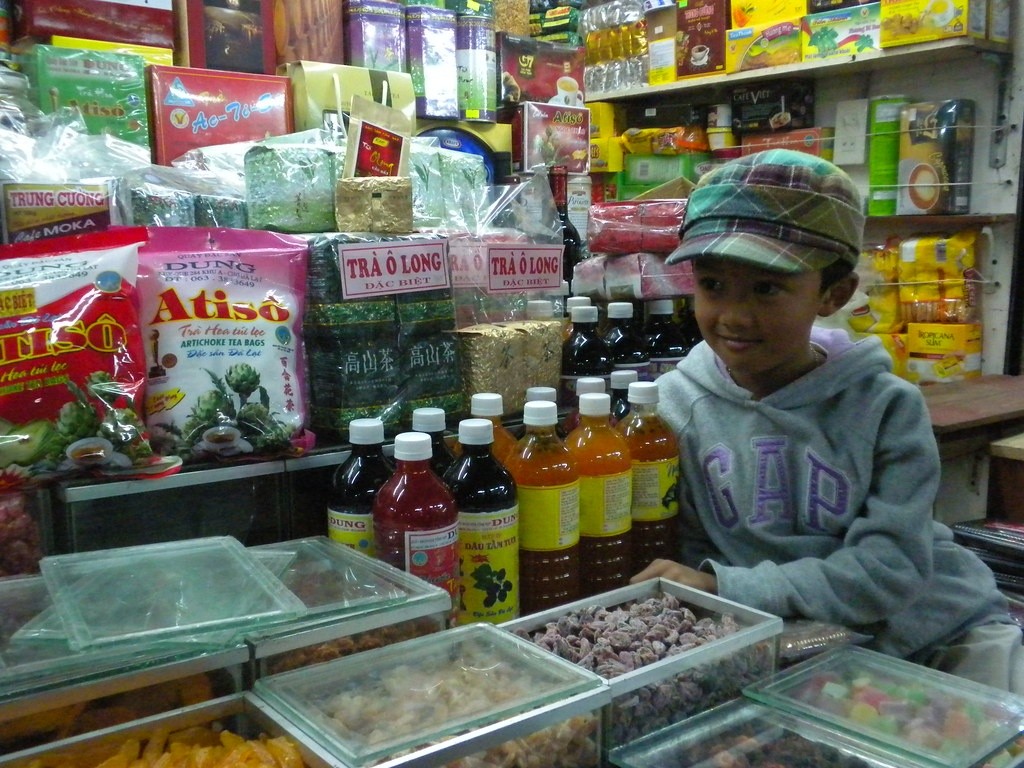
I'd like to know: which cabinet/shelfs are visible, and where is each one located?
[584,2,1024,525]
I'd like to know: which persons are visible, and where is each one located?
[622,149,1024,698]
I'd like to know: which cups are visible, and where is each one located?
[557,77,583,106]
[928,0,953,23]
[692,45,709,60]
[908,163,939,210]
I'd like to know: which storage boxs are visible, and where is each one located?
[0,406,1024,767]
[0,1,986,219]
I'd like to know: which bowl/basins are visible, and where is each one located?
[203,426,241,448]
[66,437,113,465]
[58,452,132,470]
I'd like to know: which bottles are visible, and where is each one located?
[505,401,580,612]
[517,387,569,442]
[561,304,614,407]
[647,299,689,382]
[564,393,630,596]
[328,418,395,557]
[563,296,591,341]
[443,419,521,630]
[373,431,462,632]
[527,300,552,320]
[610,370,638,422]
[565,378,617,433]
[413,408,456,477]
[458,392,517,464]
[679,297,703,346]
[614,382,680,571]
[604,300,650,381]
[548,166,581,317]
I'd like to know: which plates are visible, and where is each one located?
[691,55,708,65]
[196,439,254,453]
[897,160,927,214]
[548,96,584,108]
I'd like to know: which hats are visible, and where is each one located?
[663,148,868,274]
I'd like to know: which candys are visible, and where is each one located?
[787,666,1024,768]
